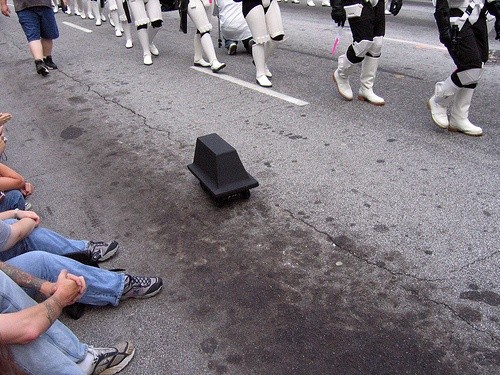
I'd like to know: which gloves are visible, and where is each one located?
[494,17,500,42]
[331,3,346,28]
[389,0,403,17]
[439,26,453,47]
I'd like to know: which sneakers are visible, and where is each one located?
[87,239,119,262]
[86,340,136,375]
[119,273,163,301]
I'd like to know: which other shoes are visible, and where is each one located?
[306,0,315,7]
[291,0,300,4]
[67,7,72,15]
[53,6,59,13]
[248,39,256,54]
[34,55,58,77]
[321,0,331,7]
[25,202,31,211]
[228,42,237,55]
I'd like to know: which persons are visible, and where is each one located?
[0,0,59,76]
[129,0,163,65]
[427,0,500,135]
[0,112,35,211]
[179,0,284,88]
[329,0,403,105]
[52,0,132,49]
[0,209,162,307]
[0,263,134,375]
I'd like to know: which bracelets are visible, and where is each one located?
[14,208,19,218]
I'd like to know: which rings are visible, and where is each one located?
[4,137,8,143]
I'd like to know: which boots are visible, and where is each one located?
[448,88,482,137]
[74,0,106,26]
[122,20,134,49]
[427,75,463,131]
[108,5,124,37]
[358,55,385,106]
[147,25,160,56]
[199,33,226,73]
[333,52,366,102]
[137,28,153,65]
[251,42,273,88]
[194,33,210,68]
